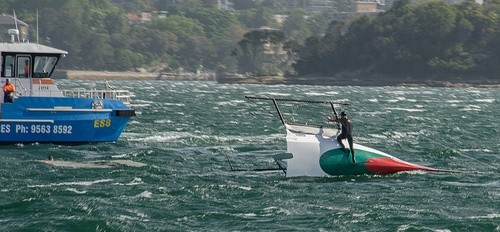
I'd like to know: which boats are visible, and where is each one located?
[1,9,137,145]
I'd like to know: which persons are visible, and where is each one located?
[327,111,356,164]
[3,78,15,103]
[23,60,28,77]
